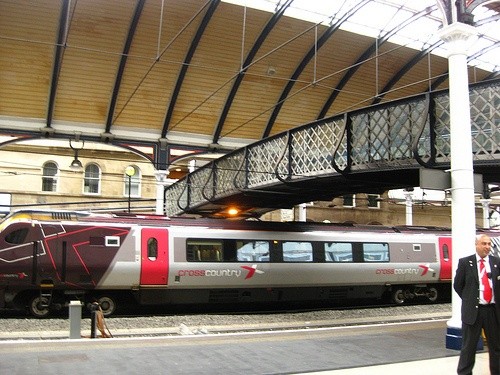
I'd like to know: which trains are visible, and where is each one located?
[0,210,500,316]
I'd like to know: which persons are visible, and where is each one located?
[454,233,500,375]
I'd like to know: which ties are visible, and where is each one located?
[479,260,492,303]
